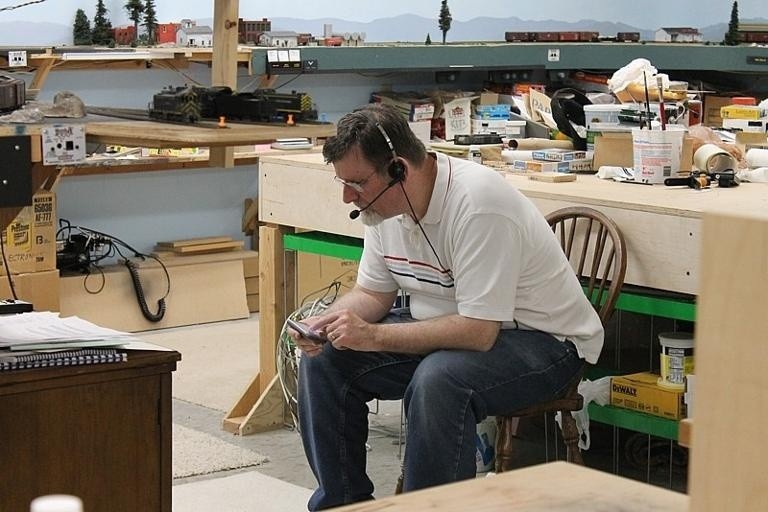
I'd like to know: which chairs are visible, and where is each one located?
[392,204,629,494]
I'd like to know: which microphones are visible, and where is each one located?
[347,184,395,220]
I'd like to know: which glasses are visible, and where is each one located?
[333,168,377,193]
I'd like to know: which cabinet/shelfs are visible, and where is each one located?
[1,37,269,178]
[0,312,182,511]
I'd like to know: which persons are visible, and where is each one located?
[287,102,605,511]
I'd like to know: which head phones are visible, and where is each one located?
[356,110,408,183]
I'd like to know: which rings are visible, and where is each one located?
[330,335,337,339]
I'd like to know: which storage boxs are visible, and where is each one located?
[371,88,559,141]
[583,95,764,169]
[603,372,687,421]
[0,187,60,275]
[0,270,61,316]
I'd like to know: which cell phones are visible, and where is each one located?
[286,318,326,346]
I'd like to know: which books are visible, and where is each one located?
[0,311,128,370]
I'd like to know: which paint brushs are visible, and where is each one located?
[613,180,653,185]
[657,77,666,130]
[639,101,643,129]
[644,71,651,130]
[644,103,649,128]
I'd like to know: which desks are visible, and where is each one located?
[217,139,767,511]
[317,456,688,512]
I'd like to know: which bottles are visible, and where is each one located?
[504,121,526,140]
